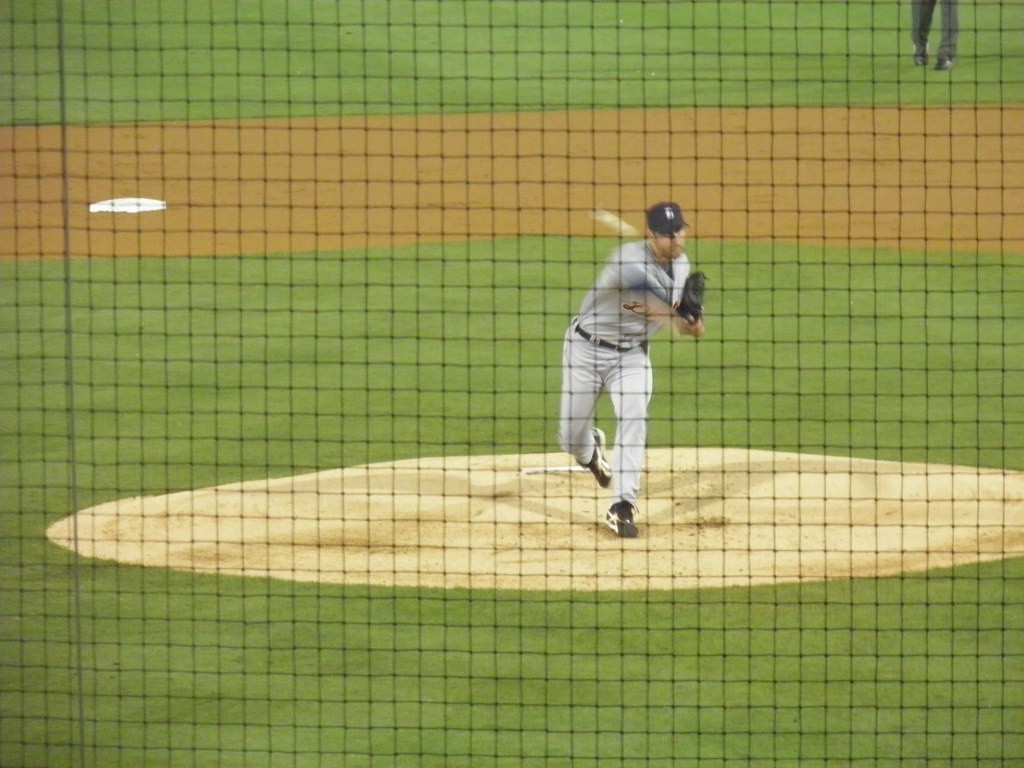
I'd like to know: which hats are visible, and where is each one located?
[646,201,690,234]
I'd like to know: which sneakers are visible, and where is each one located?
[912,40,929,67]
[933,54,957,71]
[605,503,639,538]
[586,427,615,490]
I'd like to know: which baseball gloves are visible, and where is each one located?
[677,272,706,324]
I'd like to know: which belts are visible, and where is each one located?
[576,325,645,353]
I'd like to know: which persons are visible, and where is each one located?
[911,0,958,70]
[561,200,705,537]
